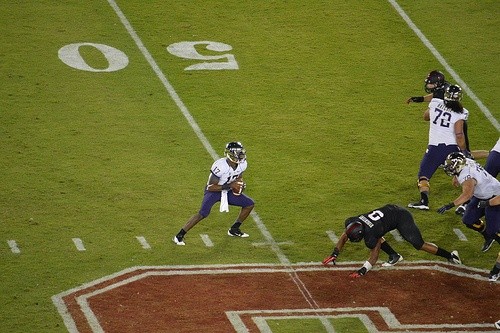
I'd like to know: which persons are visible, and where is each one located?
[323,204,461,278]
[484,136,500,178]
[438,152,500,252]
[172,142,254,245]
[408,84,474,210]
[478,195,500,329]
[408,70,491,185]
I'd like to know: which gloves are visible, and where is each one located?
[323,253,337,265]
[349,268,366,278]
[410,96,424,102]
[437,202,454,213]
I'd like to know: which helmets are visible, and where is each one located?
[443,152,466,176]
[346,221,364,242]
[424,71,445,93]
[224,141,246,163]
[444,84,463,102]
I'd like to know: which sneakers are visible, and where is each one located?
[482,238,496,252]
[228,229,250,237]
[172,236,186,245]
[382,253,403,267]
[408,202,429,210]
[450,250,462,264]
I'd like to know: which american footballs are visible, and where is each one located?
[232,177,245,196]
